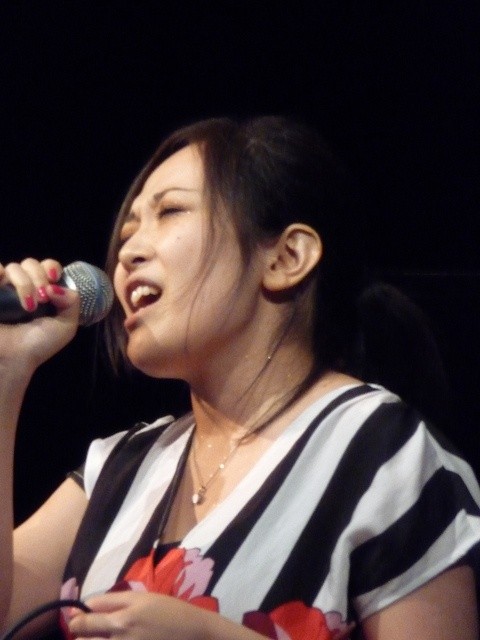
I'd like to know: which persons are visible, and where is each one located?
[0,119,480,640]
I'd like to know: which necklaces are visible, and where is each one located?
[190,421,245,505]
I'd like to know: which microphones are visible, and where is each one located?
[0,261,115,329]
